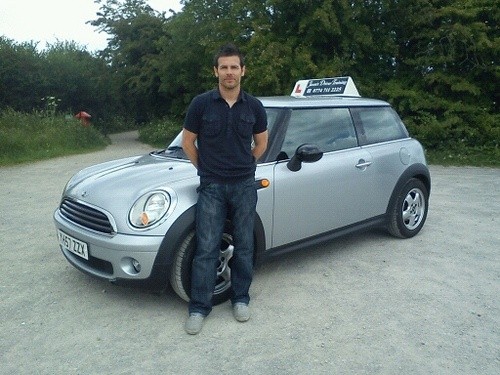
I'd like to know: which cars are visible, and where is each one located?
[53,76,431,305]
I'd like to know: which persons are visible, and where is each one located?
[182,44,269,335]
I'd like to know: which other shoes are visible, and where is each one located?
[185,313,205,335]
[234,302,249,321]
